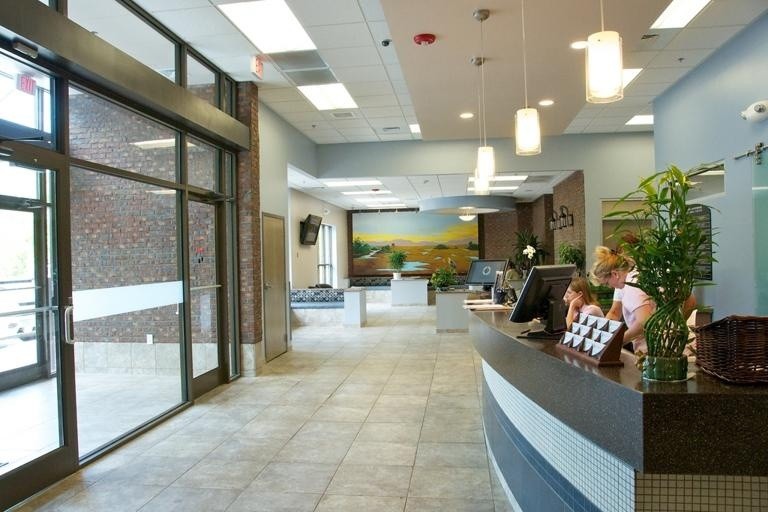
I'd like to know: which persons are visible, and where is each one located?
[602,241,697,325]
[482,259,530,306]
[590,243,698,356]
[563,276,605,331]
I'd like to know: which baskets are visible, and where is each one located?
[689,313,768,384]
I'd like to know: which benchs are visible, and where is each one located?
[289,288,344,325]
[351,274,470,306]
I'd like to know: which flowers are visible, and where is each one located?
[522,244,536,270]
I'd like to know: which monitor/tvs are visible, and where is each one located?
[509,264,576,340]
[300,214,322,245]
[464,258,509,286]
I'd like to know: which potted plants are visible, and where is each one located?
[599,164,724,383]
[389,251,408,280]
[430,265,459,291]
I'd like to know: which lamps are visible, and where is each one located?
[473,8,499,178]
[473,166,495,197]
[557,205,574,228]
[459,209,475,224]
[547,210,561,230]
[584,0,623,108]
[513,2,543,157]
